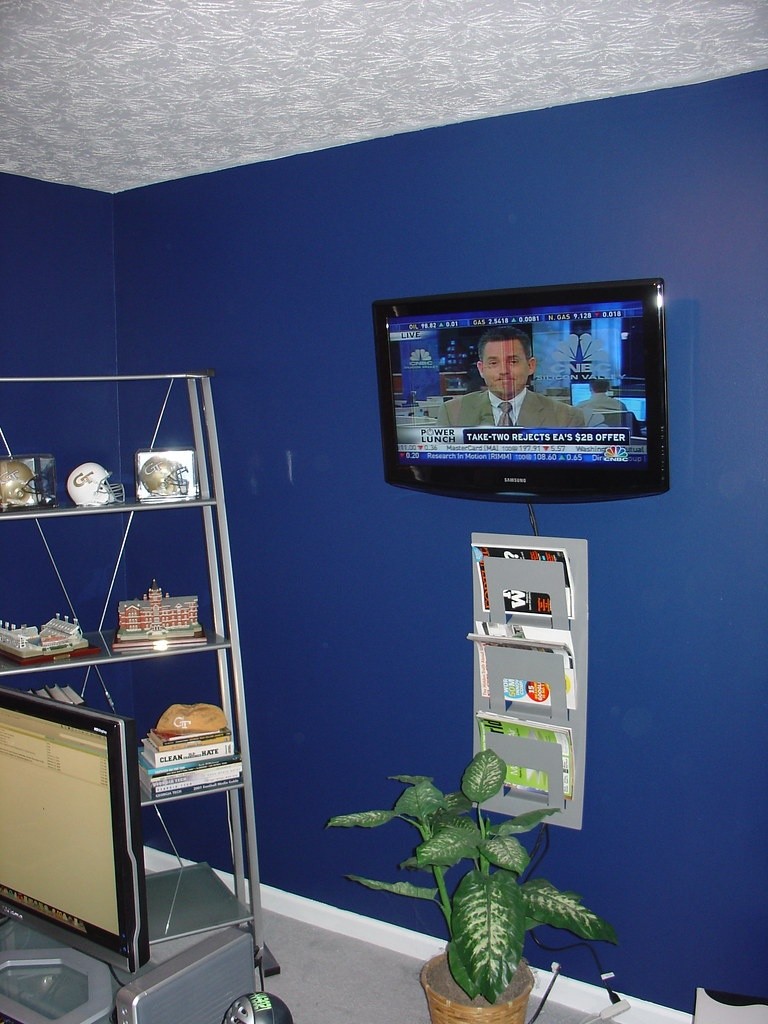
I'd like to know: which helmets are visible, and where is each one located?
[66,462,125,506]
[141,455,189,496]
[0,461,51,507]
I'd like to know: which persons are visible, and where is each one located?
[436,326,627,429]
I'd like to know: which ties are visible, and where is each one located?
[497,402,512,427]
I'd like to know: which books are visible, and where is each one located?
[0,624,243,800]
[469,543,576,802]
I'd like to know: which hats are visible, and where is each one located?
[156,703,227,735]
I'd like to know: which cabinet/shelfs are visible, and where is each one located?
[0,369,279,977]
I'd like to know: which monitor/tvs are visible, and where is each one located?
[0,688,150,1024]
[611,395,648,425]
[570,382,592,407]
[371,277,670,504]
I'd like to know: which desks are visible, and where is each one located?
[0,868,255,1024]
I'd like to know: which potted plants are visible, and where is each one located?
[322,749,618,1024]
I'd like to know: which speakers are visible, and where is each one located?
[222,992,293,1024]
